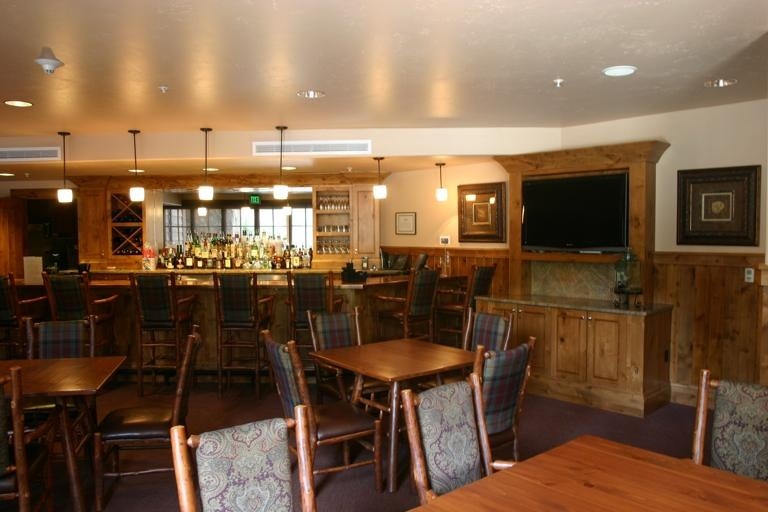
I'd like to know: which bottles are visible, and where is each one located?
[157,227,312,270]
[140,242,155,272]
[313,191,352,256]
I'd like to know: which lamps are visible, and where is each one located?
[56,132,74,203]
[435,163,448,201]
[273,127,289,200]
[373,158,387,199]
[198,128,214,201]
[128,129,145,202]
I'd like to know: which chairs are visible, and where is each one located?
[1,365,57,511]
[25,314,95,458]
[691,368,767,481]
[306,306,363,407]
[461,306,516,353]
[473,335,538,464]
[259,329,383,498]
[169,404,319,510]
[400,371,519,504]
[91,324,202,510]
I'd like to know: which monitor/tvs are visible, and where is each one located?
[521,171,628,249]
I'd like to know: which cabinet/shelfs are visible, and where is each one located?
[352,183,380,269]
[311,185,352,268]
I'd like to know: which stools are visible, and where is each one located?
[128,271,198,397]
[372,266,442,343]
[3,271,46,359]
[434,263,498,351]
[213,271,275,401]
[285,270,344,397]
[41,268,121,391]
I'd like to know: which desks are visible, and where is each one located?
[306,337,478,494]
[408,434,767,512]
[3,353,128,511]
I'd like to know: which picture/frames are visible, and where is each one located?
[676,165,762,246]
[395,212,416,235]
[457,181,507,243]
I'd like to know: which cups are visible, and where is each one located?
[361,255,369,272]
